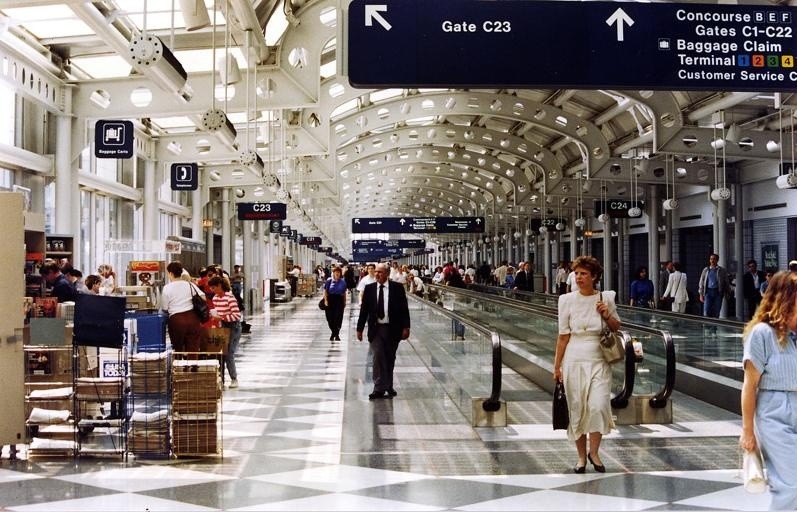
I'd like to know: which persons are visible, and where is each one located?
[628,264,655,311]
[312,260,603,308]
[36,259,245,390]
[286,264,300,297]
[551,256,622,473]
[291,265,297,269]
[356,262,413,400]
[298,266,303,276]
[317,267,347,342]
[658,253,797,319]
[736,267,797,512]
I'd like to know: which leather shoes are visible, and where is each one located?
[384,388,396,396]
[369,391,384,398]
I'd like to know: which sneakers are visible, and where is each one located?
[228,378,239,388]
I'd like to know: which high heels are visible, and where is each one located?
[587,451,606,473]
[574,460,587,474]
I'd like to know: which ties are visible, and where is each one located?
[377,284,385,319]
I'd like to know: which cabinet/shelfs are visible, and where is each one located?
[75,293,224,464]
[1,192,75,464]
[283,255,293,285]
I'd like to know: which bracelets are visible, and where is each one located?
[603,315,611,321]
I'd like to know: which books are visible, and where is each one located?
[22,353,220,458]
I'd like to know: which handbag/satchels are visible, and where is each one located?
[552,378,570,430]
[741,442,768,494]
[597,292,625,364]
[319,298,328,310]
[665,296,675,303]
[185,279,209,323]
